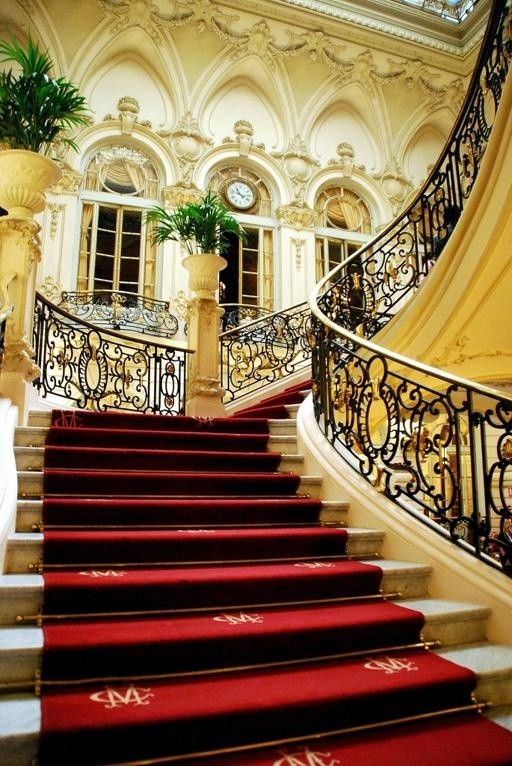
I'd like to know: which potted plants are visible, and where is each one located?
[0,23,89,220]
[140,185,250,298]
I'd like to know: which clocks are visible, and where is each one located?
[225,179,256,211]
